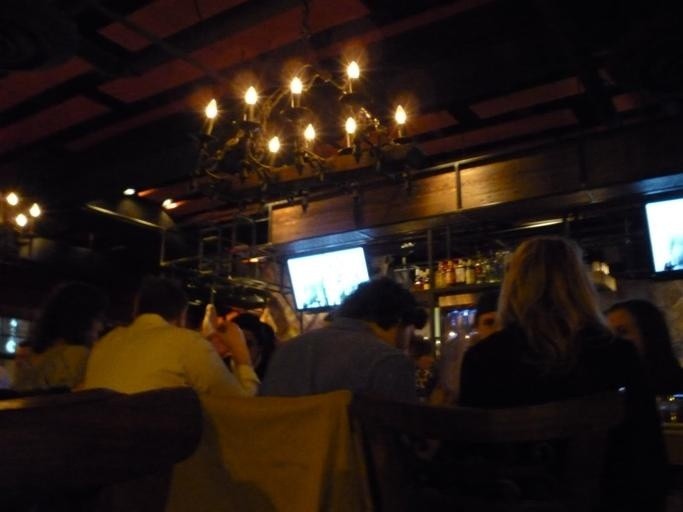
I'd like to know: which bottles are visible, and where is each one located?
[413,248,500,292]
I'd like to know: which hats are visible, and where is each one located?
[470,290,500,328]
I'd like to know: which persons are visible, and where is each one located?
[432,294,503,404]
[598,298,683,392]
[11,285,107,390]
[221,314,275,380]
[459,232,667,511]
[261,279,443,510]
[85,281,260,398]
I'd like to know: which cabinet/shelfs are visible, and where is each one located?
[262,133,613,345]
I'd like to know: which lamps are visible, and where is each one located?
[191,56,415,182]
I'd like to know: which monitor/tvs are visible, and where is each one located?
[285,245,372,313]
[636,189,683,281]
